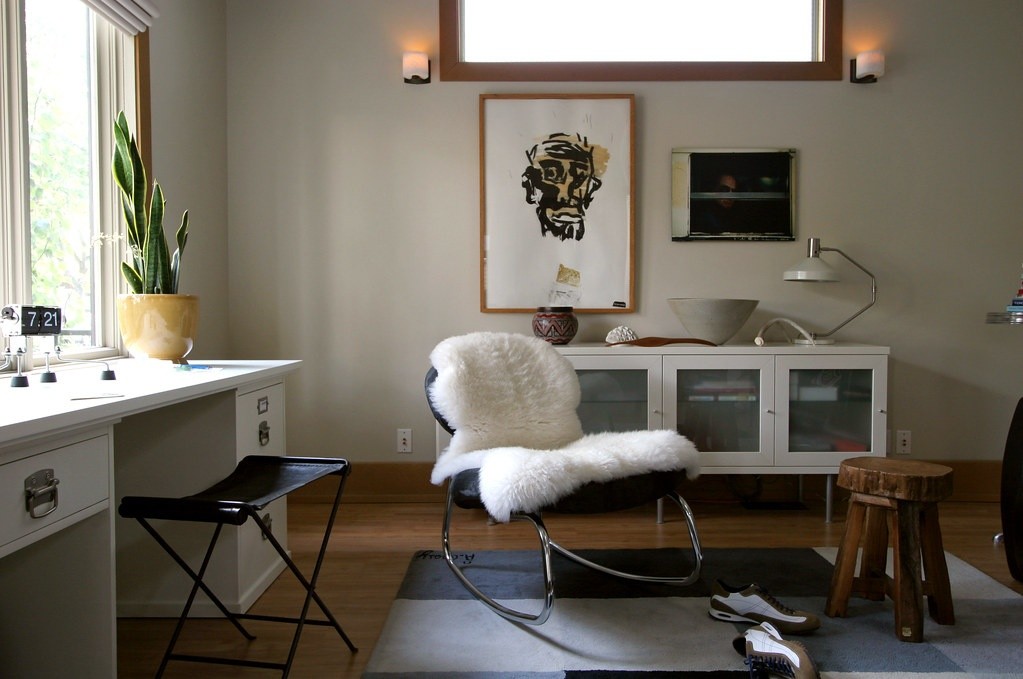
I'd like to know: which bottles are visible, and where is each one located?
[532,307,579,346]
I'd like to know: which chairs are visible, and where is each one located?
[424,332,703,624]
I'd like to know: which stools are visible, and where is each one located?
[118,455,358,679]
[825,458,954,642]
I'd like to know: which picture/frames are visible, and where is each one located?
[478,93,636,313]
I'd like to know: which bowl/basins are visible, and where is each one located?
[667,297,760,346]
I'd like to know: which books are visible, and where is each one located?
[680,381,756,401]
[1006,274,1023,312]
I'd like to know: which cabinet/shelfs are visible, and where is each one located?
[436,342,890,511]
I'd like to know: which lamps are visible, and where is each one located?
[782,238,877,345]
[849,52,886,84]
[403,52,431,84]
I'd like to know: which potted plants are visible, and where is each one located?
[111,110,200,367]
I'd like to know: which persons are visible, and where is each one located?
[691,175,741,233]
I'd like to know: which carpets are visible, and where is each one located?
[363,547,1023,679]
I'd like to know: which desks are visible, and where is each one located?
[0,357,302,679]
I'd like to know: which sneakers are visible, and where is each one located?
[733,622,821,679]
[708,581,821,635]
